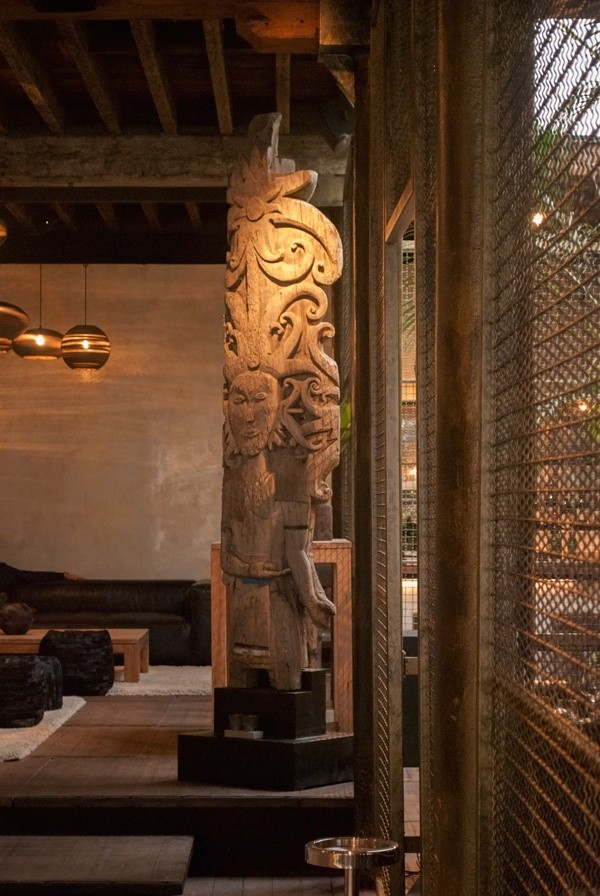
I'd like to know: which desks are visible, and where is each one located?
[211,539,351,732]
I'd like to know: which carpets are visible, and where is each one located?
[0,665,212,761]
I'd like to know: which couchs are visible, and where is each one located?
[0,580,213,670]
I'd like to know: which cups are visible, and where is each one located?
[242,715,259,731]
[228,714,242,729]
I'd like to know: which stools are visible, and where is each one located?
[0,654,62,728]
[39,629,115,696]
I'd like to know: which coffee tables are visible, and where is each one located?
[0,628,149,682]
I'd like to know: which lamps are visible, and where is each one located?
[0,218,111,370]
